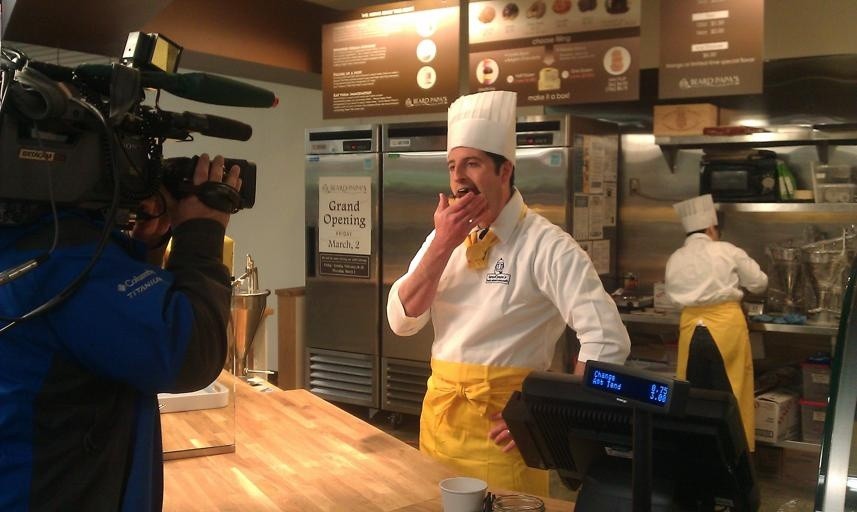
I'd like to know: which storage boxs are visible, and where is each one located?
[628,332,834,446]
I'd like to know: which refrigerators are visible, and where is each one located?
[304,114,623,418]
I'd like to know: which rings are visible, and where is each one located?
[507,430,511,437]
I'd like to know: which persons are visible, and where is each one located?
[387,89,635,508]
[0,149,244,510]
[662,194,770,455]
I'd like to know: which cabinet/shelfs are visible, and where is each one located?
[652,127,857,224]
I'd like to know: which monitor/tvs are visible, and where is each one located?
[518,370,760,512]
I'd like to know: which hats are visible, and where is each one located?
[671,194,719,234]
[445,89,519,168]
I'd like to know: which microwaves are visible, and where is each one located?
[700,157,780,206]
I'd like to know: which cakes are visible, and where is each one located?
[447,191,468,206]
[478,0,627,23]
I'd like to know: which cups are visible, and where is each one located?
[438,475,488,512]
